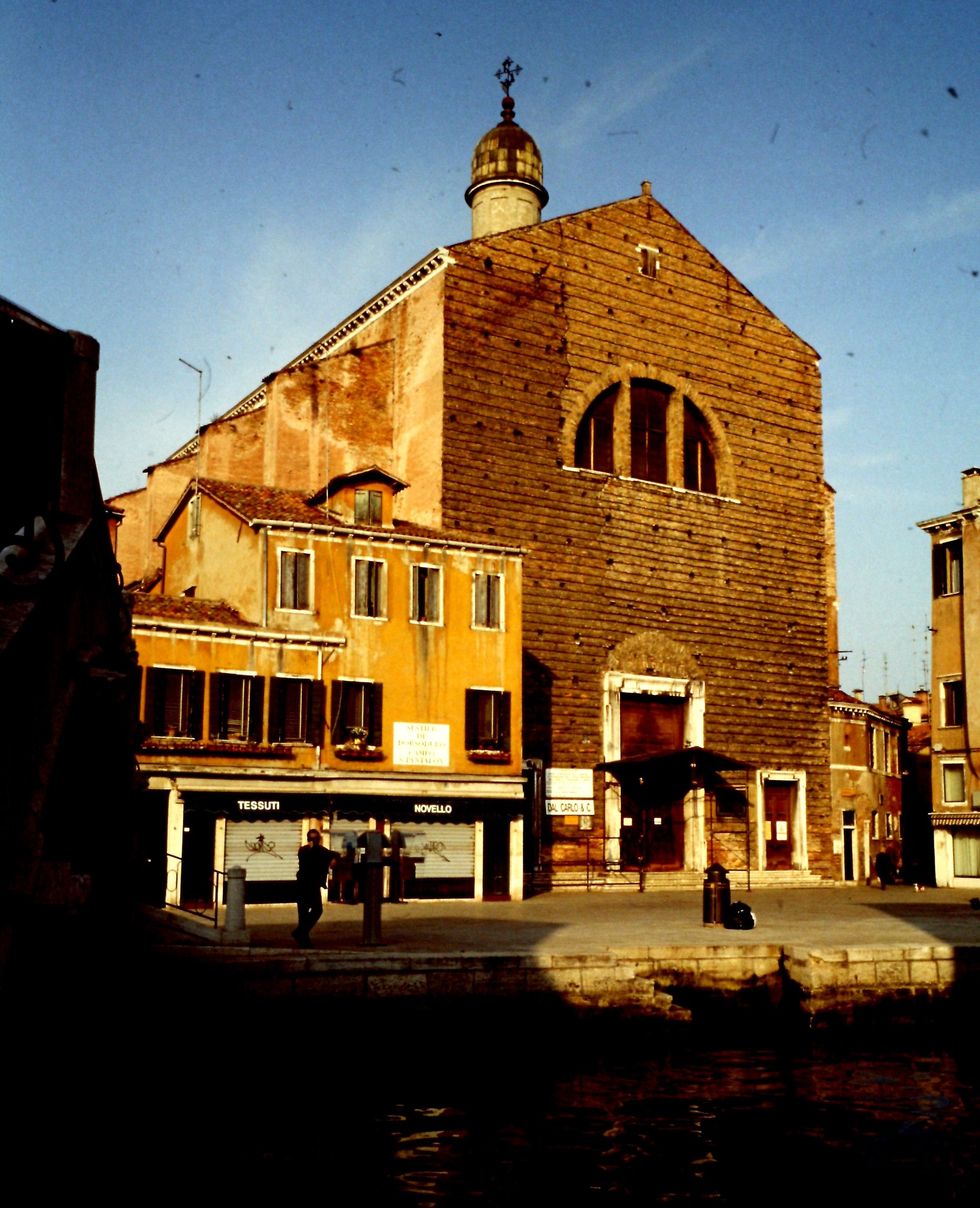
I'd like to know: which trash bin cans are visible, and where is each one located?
[702,862,731,928]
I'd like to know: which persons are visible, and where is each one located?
[875,847,893,890]
[291,829,340,948]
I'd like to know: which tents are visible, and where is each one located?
[586,747,761,892]
